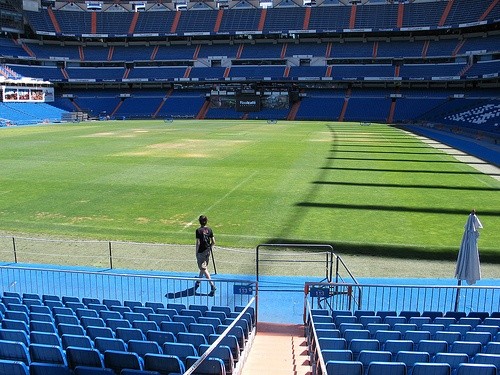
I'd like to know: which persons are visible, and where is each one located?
[191,215,216,297]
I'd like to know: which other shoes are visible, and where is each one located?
[209,286,217,296]
[195,281,200,291]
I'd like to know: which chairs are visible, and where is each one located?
[0,292,255,375]
[304,310,500,375]
[0,0,500,141]
